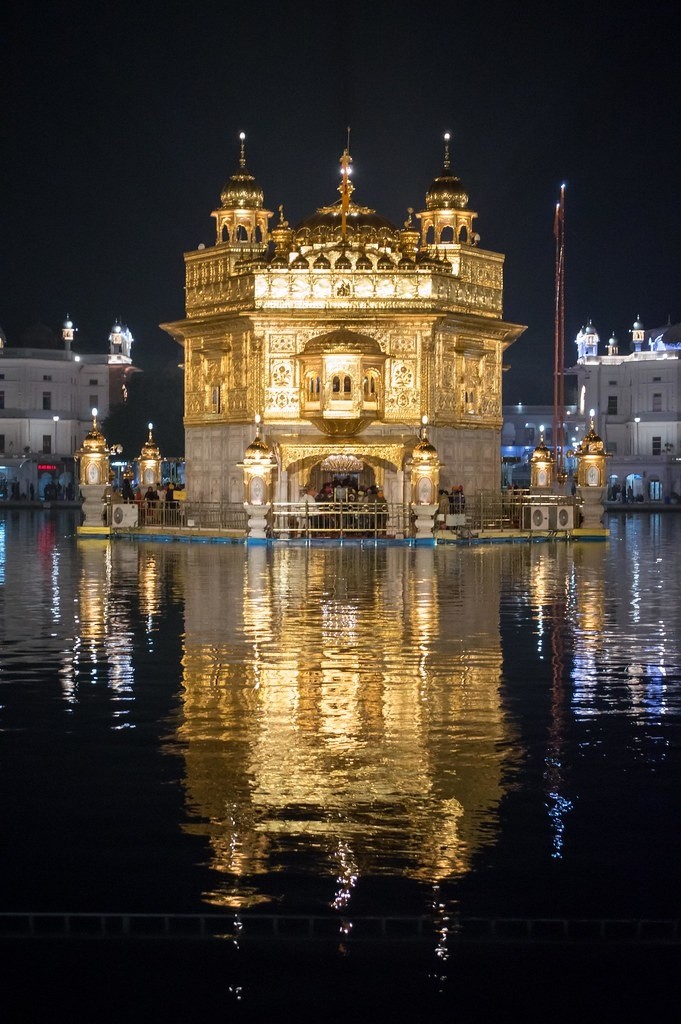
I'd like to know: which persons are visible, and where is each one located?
[613,482,636,503]
[122,479,185,526]
[294,474,389,538]
[506,480,529,499]
[437,484,466,533]
[0,476,75,502]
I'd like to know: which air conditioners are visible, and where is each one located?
[105,503,139,529]
[555,505,577,531]
[530,506,557,532]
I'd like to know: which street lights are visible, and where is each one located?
[572,406,615,530]
[137,421,161,496]
[74,408,118,528]
[526,424,553,503]
[237,411,277,540]
[406,413,445,540]
